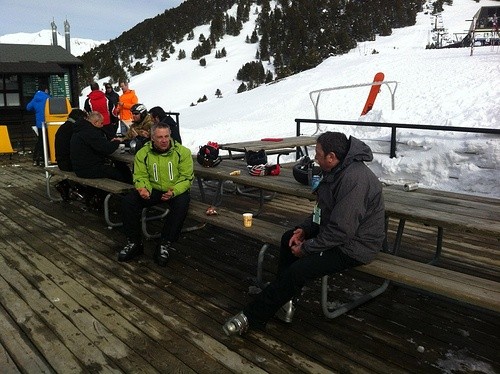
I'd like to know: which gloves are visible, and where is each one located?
[203,141,220,152]
[250,165,281,176]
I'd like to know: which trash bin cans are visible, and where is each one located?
[44,97,72,165]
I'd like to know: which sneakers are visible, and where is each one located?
[56,182,71,198]
[223,312,250,337]
[119,241,143,258]
[245,285,297,322]
[155,242,172,263]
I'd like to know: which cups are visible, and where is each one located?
[119,144,125,153]
[243,213,252,227]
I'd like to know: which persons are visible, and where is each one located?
[118,123,194,267]
[55,81,182,184]
[223,131,385,337]
[27,85,53,166]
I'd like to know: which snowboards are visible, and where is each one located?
[361,72,385,116]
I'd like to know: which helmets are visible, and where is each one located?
[294,161,322,184]
[197,144,220,166]
[130,104,148,123]
[245,150,267,170]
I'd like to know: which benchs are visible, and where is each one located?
[44,149,500,319]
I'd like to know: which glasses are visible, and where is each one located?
[131,110,139,115]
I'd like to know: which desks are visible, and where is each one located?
[106,136,500,266]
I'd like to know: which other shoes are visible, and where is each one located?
[33,161,37,168]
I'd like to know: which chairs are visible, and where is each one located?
[0,125,18,160]
